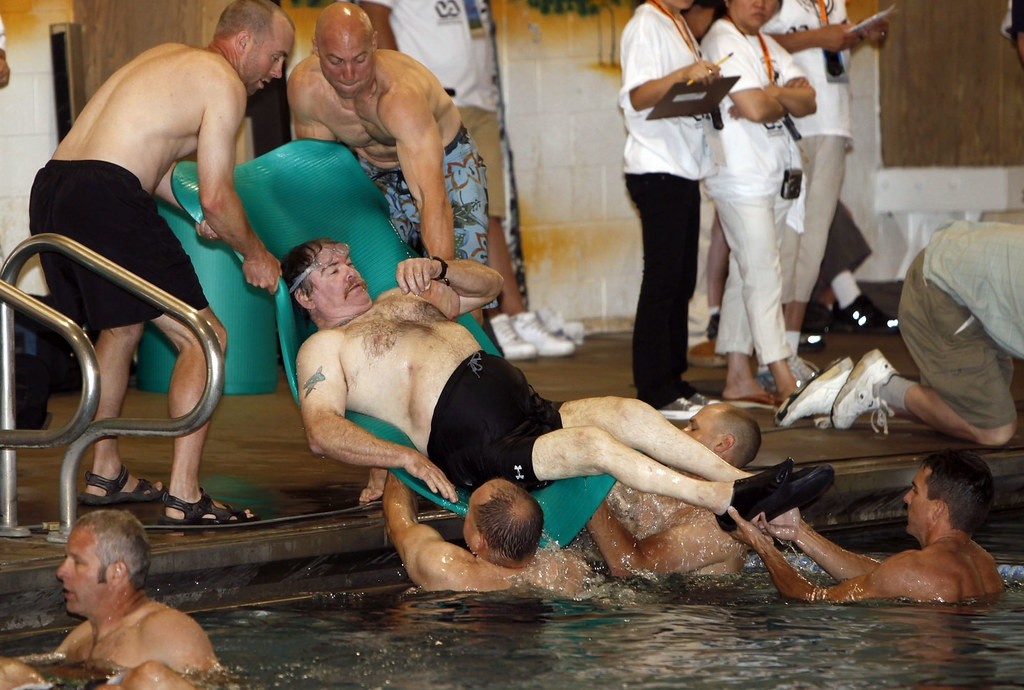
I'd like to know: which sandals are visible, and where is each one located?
[77,464,168,505]
[162,486,260,528]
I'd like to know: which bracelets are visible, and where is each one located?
[426,256,450,286]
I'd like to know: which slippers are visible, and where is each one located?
[772,400,828,424]
[724,385,778,409]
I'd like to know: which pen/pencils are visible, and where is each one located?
[685,50,734,87]
[844,19,864,41]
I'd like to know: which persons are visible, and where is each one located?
[774,219,1024,446]
[381,404,1003,601]
[281,237,833,533]
[29,0,296,526]
[0,511,219,690]
[370,0,575,361]
[0,18,9,89]
[1001,0,1024,64]
[288,2,489,503]
[618,0,903,421]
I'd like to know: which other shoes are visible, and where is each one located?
[765,464,834,517]
[830,292,899,334]
[708,315,719,340]
[756,356,820,392]
[712,457,794,532]
[798,333,826,350]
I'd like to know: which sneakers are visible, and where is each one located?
[657,397,703,420]
[830,348,900,434]
[689,394,722,405]
[510,311,575,358]
[489,313,538,360]
[775,356,854,427]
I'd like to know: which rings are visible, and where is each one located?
[881,31,886,36]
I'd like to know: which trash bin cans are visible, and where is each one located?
[138,197,280,394]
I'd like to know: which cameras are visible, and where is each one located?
[781,168,802,199]
